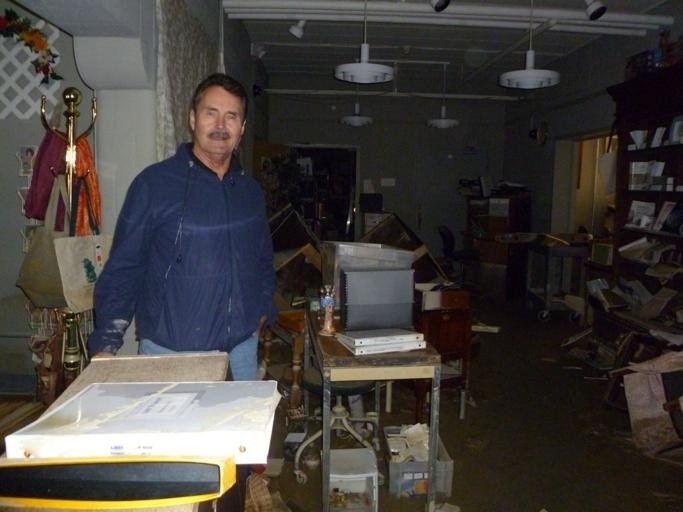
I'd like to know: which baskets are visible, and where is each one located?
[244,472,272,512]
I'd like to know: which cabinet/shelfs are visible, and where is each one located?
[582,108,683,350]
[413,282,474,424]
[467,191,534,265]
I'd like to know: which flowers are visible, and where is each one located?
[0,6,64,87]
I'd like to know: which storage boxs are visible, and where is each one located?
[383,424,453,498]
[319,449,379,512]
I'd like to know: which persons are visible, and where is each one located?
[86,72,277,383]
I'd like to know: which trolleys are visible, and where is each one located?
[516,242,590,324]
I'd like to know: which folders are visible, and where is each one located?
[334,329,427,356]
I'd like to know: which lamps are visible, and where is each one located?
[426,63,458,129]
[585,0,608,21]
[334,0,395,85]
[339,59,374,127]
[288,19,307,39]
[429,0,451,12]
[500,0,564,88]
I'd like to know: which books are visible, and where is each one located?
[331,327,425,346]
[334,336,428,355]
[4,383,281,465]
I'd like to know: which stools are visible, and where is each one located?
[294,365,375,487]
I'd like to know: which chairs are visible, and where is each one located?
[437,224,481,282]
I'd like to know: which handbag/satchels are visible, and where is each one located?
[15,174,81,307]
[54,176,123,314]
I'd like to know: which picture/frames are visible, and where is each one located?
[590,239,620,273]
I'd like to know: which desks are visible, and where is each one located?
[0,351,232,512]
[468,229,607,324]
[302,289,441,512]
